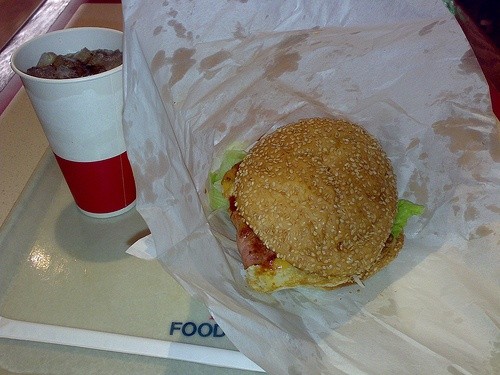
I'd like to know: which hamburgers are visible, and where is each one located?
[207,116,425,294]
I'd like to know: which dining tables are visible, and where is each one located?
[1,1,500,375]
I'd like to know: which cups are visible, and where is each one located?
[9,25,139,218]
